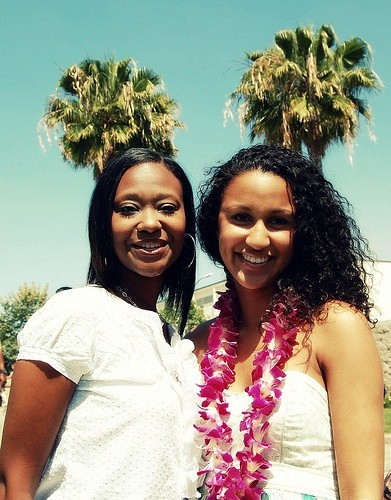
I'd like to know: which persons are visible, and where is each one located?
[0,147,203,500]
[182,142,385,500]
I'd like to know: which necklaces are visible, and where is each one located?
[193,281,311,500]
[115,284,139,309]
